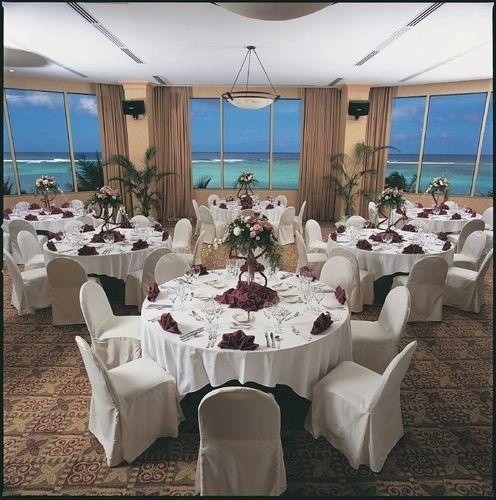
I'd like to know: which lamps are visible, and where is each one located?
[223,45,281,110]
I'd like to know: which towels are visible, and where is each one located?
[471,210,477,217]
[61,202,69,208]
[147,283,159,302]
[402,224,417,232]
[218,330,258,350]
[63,211,73,217]
[77,246,98,256]
[416,203,423,208]
[219,204,227,209]
[158,313,181,334]
[438,232,447,240]
[442,241,451,251]
[213,200,216,205]
[356,239,372,251]
[131,239,149,250]
[465,208,473,213]
[362,222,375,228]
[155,224,162,232]
[311,313,333,335]
[47,241,57,251]
[417,212,428,218]
[401,245,425,254]
[80,225,94,232]
[335,286,346,305]
[330,231,337,241]
[25,214,37,221]
[450,213,461,219]
[265,204,274,209]
[162,232,170,241]
[4,209,12,213]
[3,213,9,220]
[337,225,345,233]
[188,264,208,275]
[297,267,316,281]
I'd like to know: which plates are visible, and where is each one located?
[212,282,226,288]
[270,283,290,291]
[232,312,253,323]
[283,296,298,303]
[195,293,211,300]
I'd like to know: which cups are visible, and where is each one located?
[183,266,192,289]
[272,304,287,334]
[362,219,370,231]
[225,259,232,280]
[175,288,187,312]
[381,233,390,250]
[203,306,215,333]
[109,233,115,250]
[385,234,394,251]
[167,287,177,312]
[313,289,325,312]
[133,220,156,243]
[303,285,315,313]
[102,233,109,249]
[212,299,222,329]
[262,302,276,331]
[192,266,201,289]
[65,224,85,250]
[232,258,241,279]
[346,225,362,247]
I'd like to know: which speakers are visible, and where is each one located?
[348,100,371,117]
[122,99,145,115]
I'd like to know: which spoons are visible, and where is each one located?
[275,335,282,349]
[291,326,311,341]
[190,310,204,323]
[264,331,282,349]
[230,322,252,330]
[205,332,217,348]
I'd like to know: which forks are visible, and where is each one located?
[149,317,160,322]
[102,248,112,254]
[180,326,204,342]
[147,303,172,312]
[175,277,191,287]
[279,311,300,323]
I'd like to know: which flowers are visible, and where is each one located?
[424,177,452,214]
[83,186,132,241]
[222,212,281,310]
[33,174,63,214]
[233,168,259,206]
[376,187,408,244]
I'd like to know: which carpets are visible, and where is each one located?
[2,219,495,497]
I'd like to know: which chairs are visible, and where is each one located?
[242,209,255,216]
[274,195,287,208]
[13,202,28,214]
[192,199,201,234]
[154,253,190,286]
[195,205,229,244]
[305,218,328,254]
[391,257,448,321]
[454,231,486,271]
[3,249,51,316]
[130,215,150,228]
[294,230,326,278]
[196,386,287,496]
[275,206,295,246]
[443,247,493,313]
[346,216,369,228]
[186,230,206,272]
[294,200,306,236]
[208,194,221,208]
[64,219,86,234]
[367,202,389,229]
[125,247,171,313]
[319,257,354,312]
[304,341,416,473]
[320,248,374,312]
[79,281,141,368]
[350,286,410,374]
[70,200,83,209]
[46,257,104,326]
[3,208,13,220]
[172,218,193,253]
[73,336,179,468]
[482,207,493,230]
